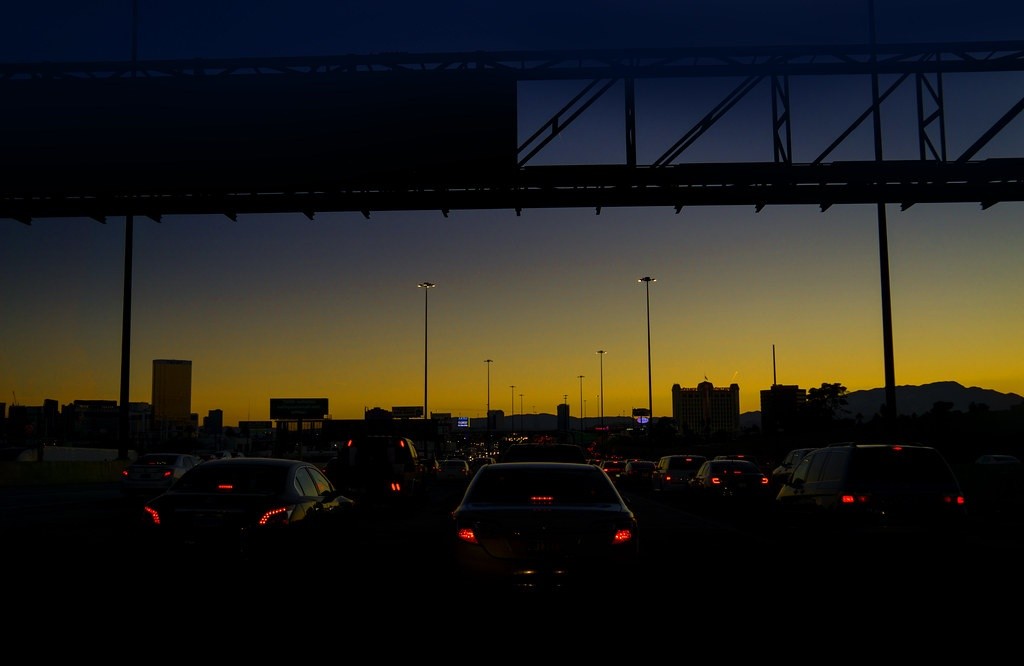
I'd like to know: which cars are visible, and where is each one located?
[132,456,367,586]
[446,461,641,602]
[122,452,205,504]
[214,432,1024,520]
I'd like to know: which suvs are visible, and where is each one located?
[755,440,975,592]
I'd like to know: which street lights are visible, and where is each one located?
[638,276,658,433]
[519,394,524,433]
[419,282,436,455]
[596,350,608,452]
[483,359,494,458]
[509,385,516,436]
[577,375,586,447]
